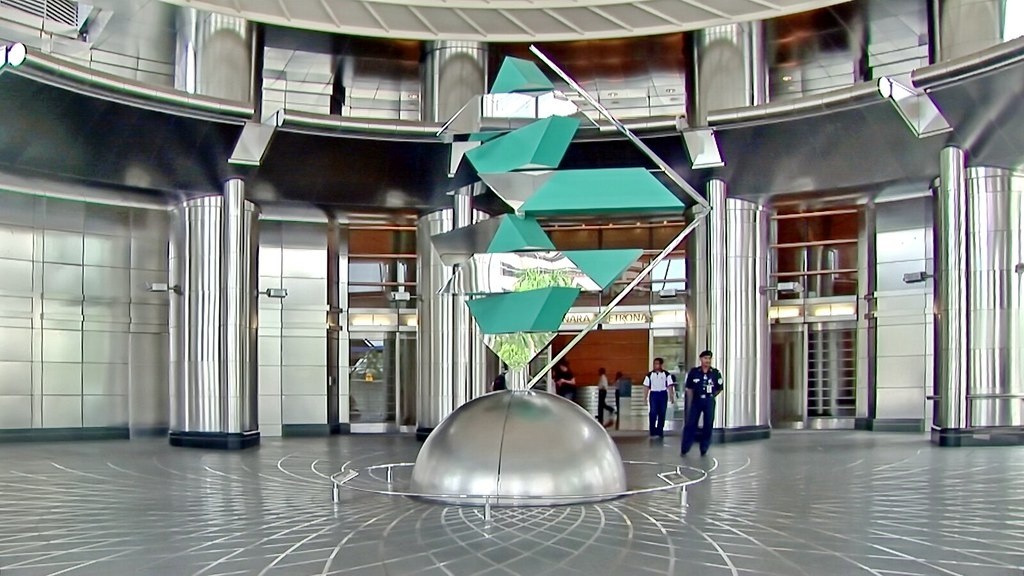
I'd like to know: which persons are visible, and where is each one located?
[682,351,723,456]
[614,372,623,413]
[552,361,576,400]
[595,368,614,417]
[641,358,679,439]
[493,361,508,391]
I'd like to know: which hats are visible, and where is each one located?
[699,350,712,357]
